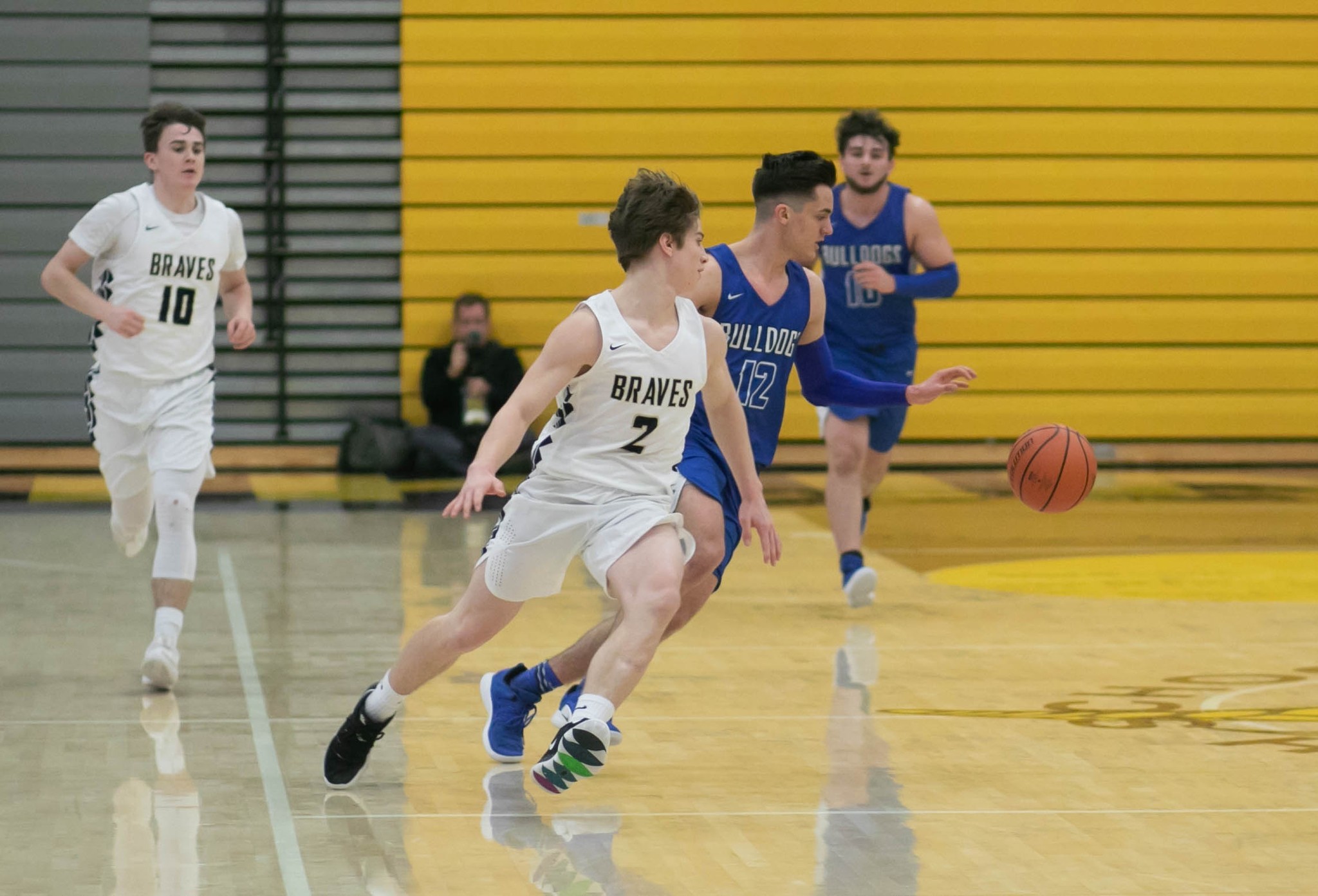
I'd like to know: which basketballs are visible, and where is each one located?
[1007,421,1098,513]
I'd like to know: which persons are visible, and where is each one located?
[479,148,976,762]
[323,167,780,794]
[798,107,961,611]
[422,291,538,475]
[44,103,254,689]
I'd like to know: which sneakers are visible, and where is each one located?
[141,692,180,745]
[480,661,542,762]
[836,628,877,697]
[115,777,154,861]
[551,683,623,746]
[528,811,629,896]
[323,793,393,881]
[840,554,875,607]
[859,496,872,536]
[142,640,181,691]
[481,764,539,849]
[531,717,610,795]
[323,682,398,789]
[114,526,148,556]
